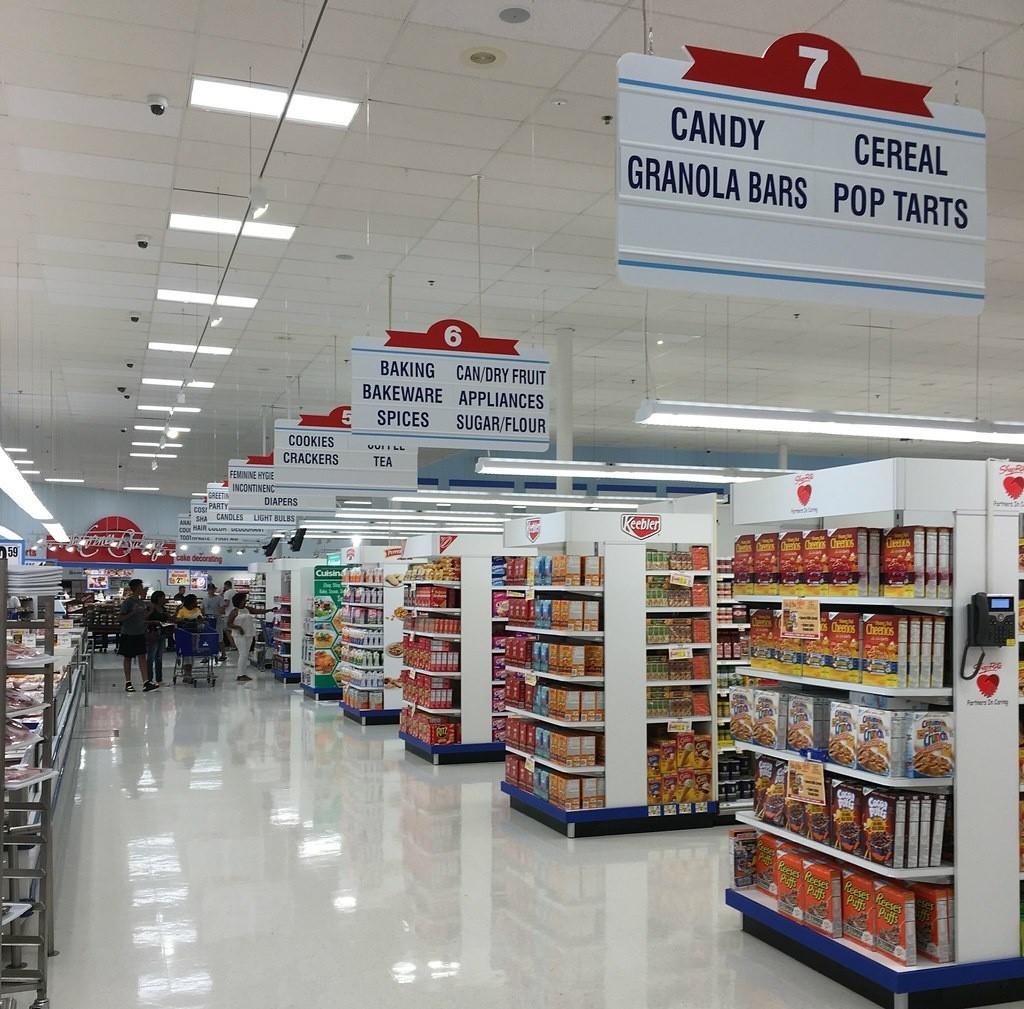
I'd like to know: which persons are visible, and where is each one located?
[227,592,278,681]
[7,595,21,609]
[117,579,161,693]
[140,580,239,686]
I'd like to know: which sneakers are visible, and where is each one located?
[125,682,135,692]
[142,681,160,692]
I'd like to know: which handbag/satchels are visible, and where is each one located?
[144,629,157,644]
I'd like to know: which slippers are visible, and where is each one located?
[219,656,228,661]
[200,659,207,663]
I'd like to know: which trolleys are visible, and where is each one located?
[173,620,219,687]
[257,615,277,672]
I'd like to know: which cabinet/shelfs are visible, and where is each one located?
[398,534,538,765]
[300,553,347,701]
[338,546,411,727]
[273,559,327,685]
[500,510,765,837]
[724,457,1024,1009]
[0,547,61,1009]
[248,561,290,670]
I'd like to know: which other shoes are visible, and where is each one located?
[156,680,170,687]
[182,676,194,684]
[236,675,252,681]
[226,645,236,651]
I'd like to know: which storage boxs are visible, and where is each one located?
[247,523,957,968]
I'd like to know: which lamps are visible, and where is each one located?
[163,423,170,434]
[209,185,224,327]
[248,67,269,220]
[273,495,671,540]
[27,532,176,557]
[152,458,158,471]
[226,546,233,552]
[254,548,259,552]
[238,547,245,555]
[475,450,812,485]
[177,390,185,403]
[185,261,199,385]
[160,435,166,449]
[633,287,1024,446]
[0,442,70,542]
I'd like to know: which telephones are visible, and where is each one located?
[967,593,1016,646]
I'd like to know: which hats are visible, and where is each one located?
[207,583,217,589]
